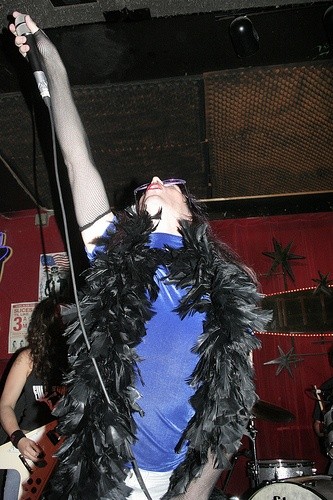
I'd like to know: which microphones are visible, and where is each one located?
[15,14,52,107]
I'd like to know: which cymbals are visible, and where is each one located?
[253,401,296,423]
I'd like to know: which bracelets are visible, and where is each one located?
[10,429,26,449]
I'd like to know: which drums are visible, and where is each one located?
[323,407,333,458]
[248,475,333,500]
[247,459,316,492]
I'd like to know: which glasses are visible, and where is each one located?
[134,178,191,206]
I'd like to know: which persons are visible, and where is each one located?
[0,297,66,463]
[9,11,253,500]
[311,346,333,476]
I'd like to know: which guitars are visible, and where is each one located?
[0,419,68,500]
